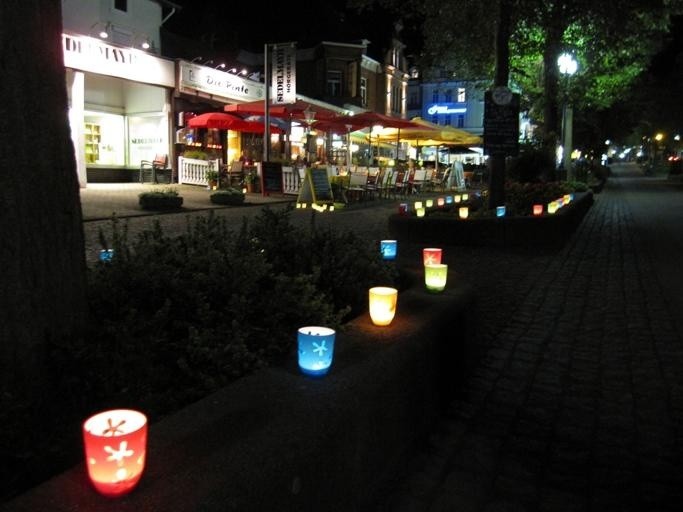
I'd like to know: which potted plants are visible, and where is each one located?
[205,171,219,189]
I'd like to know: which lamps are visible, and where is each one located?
[131,32,150,50]
[190,56,255,79]
[87,22,108,39]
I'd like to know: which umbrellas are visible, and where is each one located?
[188,98,483,166]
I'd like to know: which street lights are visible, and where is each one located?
[302,105,316,167]
[555,50,577,181]
[603,138,611,165]
[372,125,383,167]
[641,133,680,168]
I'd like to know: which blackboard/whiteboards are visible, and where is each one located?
[454,161,465,189]
[307,166,335,204]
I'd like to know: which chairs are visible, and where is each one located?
[139,153,168,184]
[219,161,452,209]
